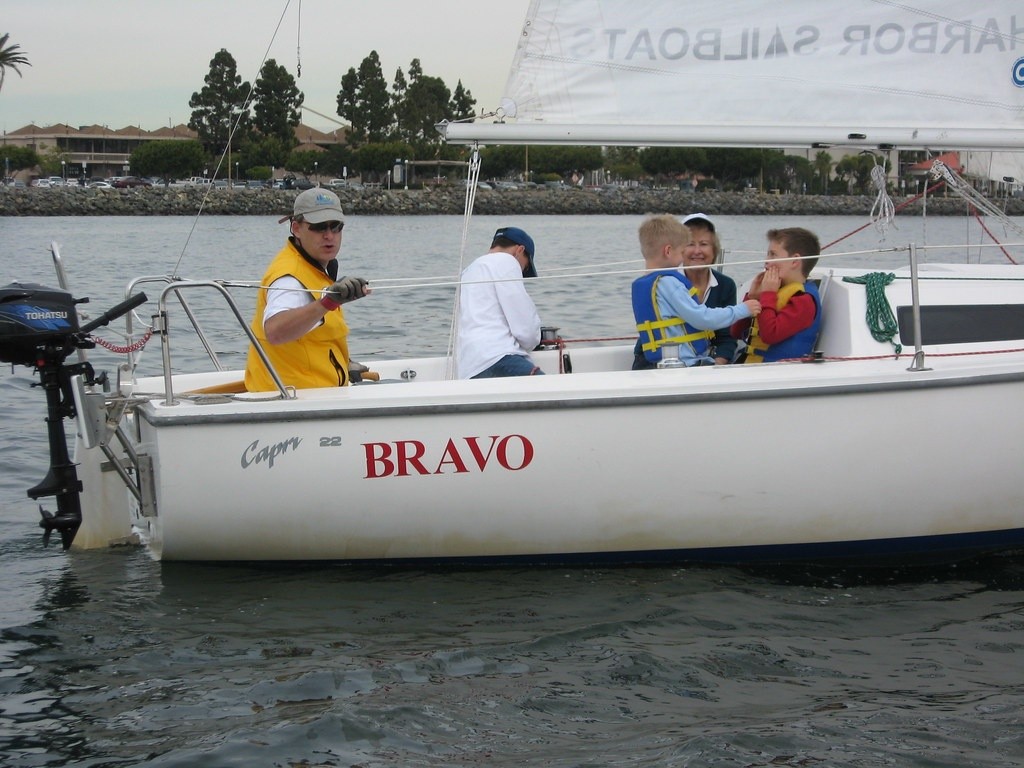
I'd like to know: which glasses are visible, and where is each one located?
[300,221,344,233]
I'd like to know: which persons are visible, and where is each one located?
[244,187,405,393]
[455,227,548,380]
[631,213,823,371]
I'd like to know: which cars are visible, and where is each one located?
[330,178,345,190]
[350,182,364,190]
[495,178,567,192]
[108,174,317,191]
[85,181,115,189]
[469,182,492,193]
[2,172,90,189]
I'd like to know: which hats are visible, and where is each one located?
[682,212,715,233]
[495,226,538,277]
[278,186,346,223]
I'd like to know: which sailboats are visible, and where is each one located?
[0,0,1024,573]
